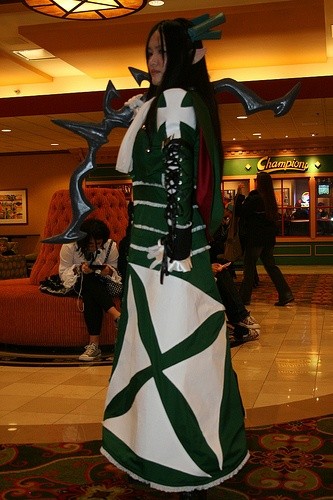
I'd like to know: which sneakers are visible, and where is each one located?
[79,345,101,361]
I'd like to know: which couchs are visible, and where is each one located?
[0,253,28,279]
[0,188,129,346]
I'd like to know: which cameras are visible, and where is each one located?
[225,217,230,225]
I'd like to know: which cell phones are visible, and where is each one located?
[89,265,107,269]
[221,262,231,267]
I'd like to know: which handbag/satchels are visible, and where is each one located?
[106,277,123,294]
[223,312,260,346]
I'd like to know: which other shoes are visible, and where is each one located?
[274,297,294,307]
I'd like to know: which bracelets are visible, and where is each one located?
[76,265,82,274]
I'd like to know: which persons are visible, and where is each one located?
[208,261,260,342]
[98,12,254,495]
[233,172,295,306]
[58,218,123,362]
[233,172,330,230]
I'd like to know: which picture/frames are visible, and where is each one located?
[274,188,290,206]
[0,188,29,226]
